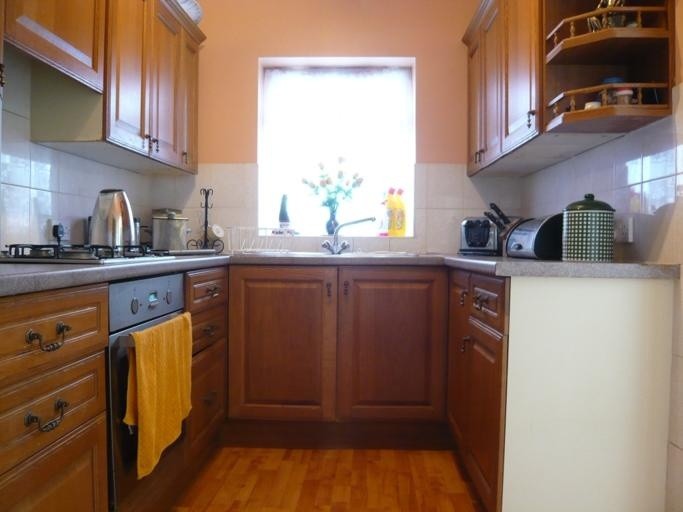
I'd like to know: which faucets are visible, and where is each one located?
[334,217,377,245]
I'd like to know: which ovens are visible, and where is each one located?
[107,273,192,510]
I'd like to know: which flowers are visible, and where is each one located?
[306,159,361,204]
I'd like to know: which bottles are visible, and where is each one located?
[563,192,614,264]
[385,187,405,235]
[586,77,633,109]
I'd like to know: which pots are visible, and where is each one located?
[151,217,189,250]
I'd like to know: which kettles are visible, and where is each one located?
[88,189,136,251]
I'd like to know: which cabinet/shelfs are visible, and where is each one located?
[27,0,180,174]
[0,283,110,510]
[538,5,673,134]
[448,269,673,510]
[228,265,447,401]
[0,1,104,92]
[465,0,501,177]
[182,31,198,171]
[185,266,230,477]
[502,1,628,176]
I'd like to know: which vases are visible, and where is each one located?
[326,199,337,233]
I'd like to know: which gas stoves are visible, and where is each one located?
[1,243,176,264]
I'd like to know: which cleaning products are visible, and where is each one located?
[378,188,394,237]
[386,190,406,236]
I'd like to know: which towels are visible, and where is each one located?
[123,310,193,481]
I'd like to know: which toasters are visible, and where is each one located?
[458,216,503,255]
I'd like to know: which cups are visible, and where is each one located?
[203,225,225,241]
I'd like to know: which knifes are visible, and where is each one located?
[484,203,510,231]
